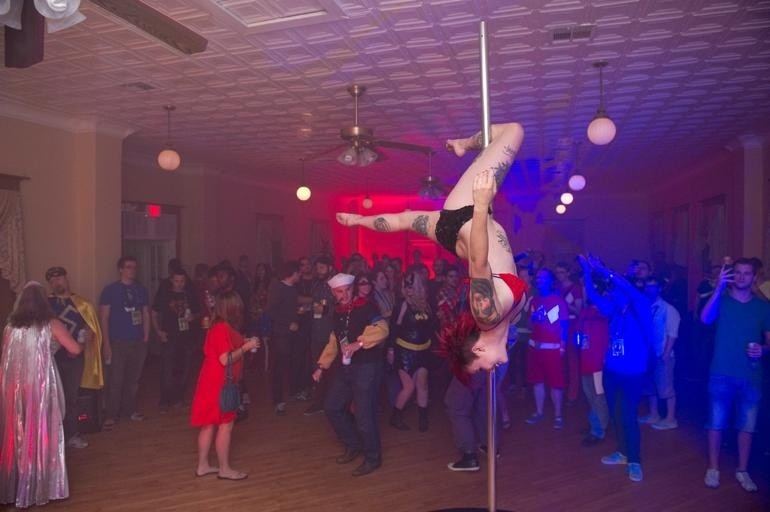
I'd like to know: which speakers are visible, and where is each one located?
[5,0,44,68]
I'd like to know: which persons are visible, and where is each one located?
[257,252,682,482]
[337,121,531,390]
[4,253,263,509]
[623,252,770,491]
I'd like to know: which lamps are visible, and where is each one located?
[0,1,87,35]
[153,104,183,173]
[361,178,373,210]
[296,157,312,204]
[584,62,617,147]
[337,139,379,168]
[566,141,587,192]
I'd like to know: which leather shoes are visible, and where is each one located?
[336,445,360,464]
[583,434,604,446]
[352,456,381,477]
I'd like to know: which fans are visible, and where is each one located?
[0,0,208,69]
[298,84,436,161]
[409,150,455,200]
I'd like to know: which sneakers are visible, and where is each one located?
[526,411,544,425]
[129,412,147,421]
[296,390,313,399]
[553,416,566,431]
[703,468,721,489]
[68,435,89,450]
[303,402,326,416]
[626,462,643,483]
[276,401,287,417]
[601,452,628,465]
[734,470,759,494]
[651,417,678,432]
[447,452,481,473]
[638,414,660,425]
[478,441,501,457]
[102,418,117,430]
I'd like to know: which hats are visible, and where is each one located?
[326,272,356,289]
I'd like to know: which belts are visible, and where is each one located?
[529,339,562,351]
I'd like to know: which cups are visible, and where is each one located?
[342,349,352,365]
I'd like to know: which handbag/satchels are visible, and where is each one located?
[220,380,242,411]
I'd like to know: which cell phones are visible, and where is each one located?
[722,264,735,285]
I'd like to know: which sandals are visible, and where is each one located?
[217,469,249,480]
[195,464,219,477]
[501,417,512,434]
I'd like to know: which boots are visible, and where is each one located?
[415,405,430,431]
[392,406,412,432]
